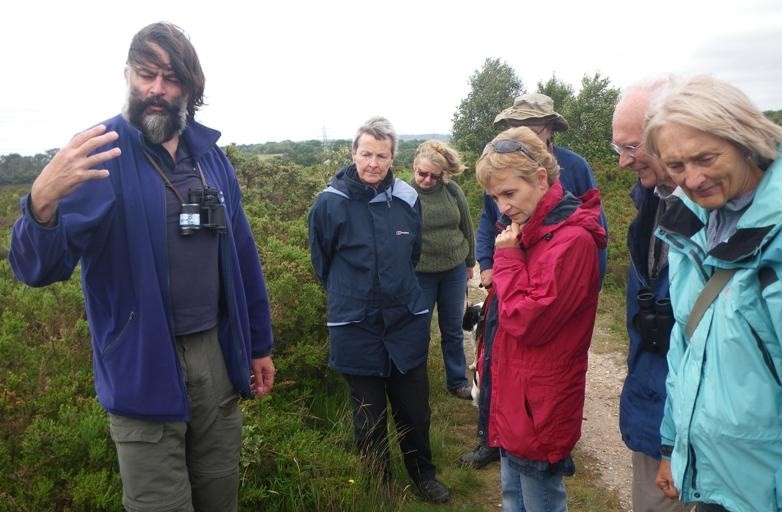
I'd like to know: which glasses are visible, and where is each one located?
[481,137,543,168]
[416,166,441,181]
[608,140,646,158]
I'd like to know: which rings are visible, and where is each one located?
[506,229,512,233]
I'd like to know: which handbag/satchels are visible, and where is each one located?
[462,301,484,331]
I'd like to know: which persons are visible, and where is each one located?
[459,90,609,468]
[648,79,782,512]
[476,126,604,512]
[9,23,273,512]
[410,136,474,403]
[609,83,707,511]
[305,116,454,504]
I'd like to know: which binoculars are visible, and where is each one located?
[462,305,481,332]
[177,187,227,236]
[636,293,676,356]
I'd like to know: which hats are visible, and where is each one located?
[491,90,571,134]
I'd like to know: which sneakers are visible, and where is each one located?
[414,476,452,504]
[456,442,499,471]
[451,384,473,400]
